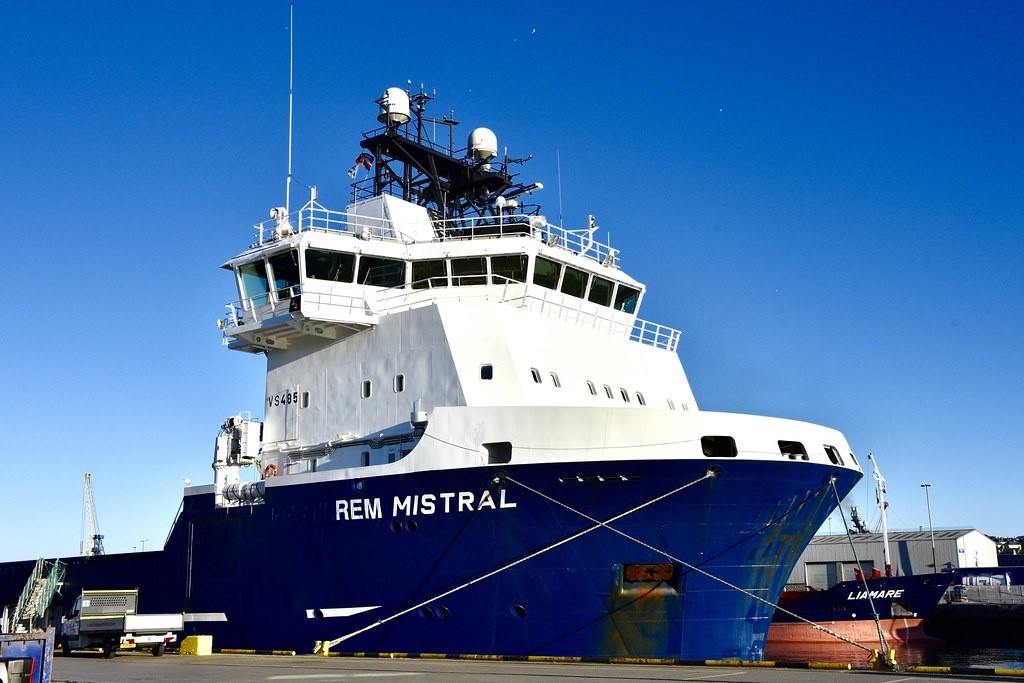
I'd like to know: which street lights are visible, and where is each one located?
[921,484,937,574]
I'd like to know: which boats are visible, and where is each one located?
[0,5,864,666]
[764,454,958,670]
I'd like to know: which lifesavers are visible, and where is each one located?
[264,464,278,479]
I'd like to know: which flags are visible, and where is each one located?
[355,152,374,170]
[348,164,359,179]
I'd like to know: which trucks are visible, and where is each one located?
[60,588,185,659]
